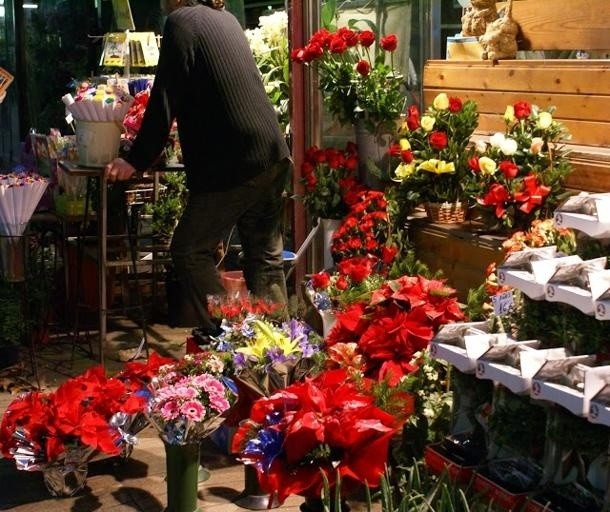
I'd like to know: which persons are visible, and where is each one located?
[0,90,6,104]
[102,1,294,325]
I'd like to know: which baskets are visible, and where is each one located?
[424,197,470,224]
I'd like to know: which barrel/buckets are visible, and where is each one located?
[72,120,122,168]
